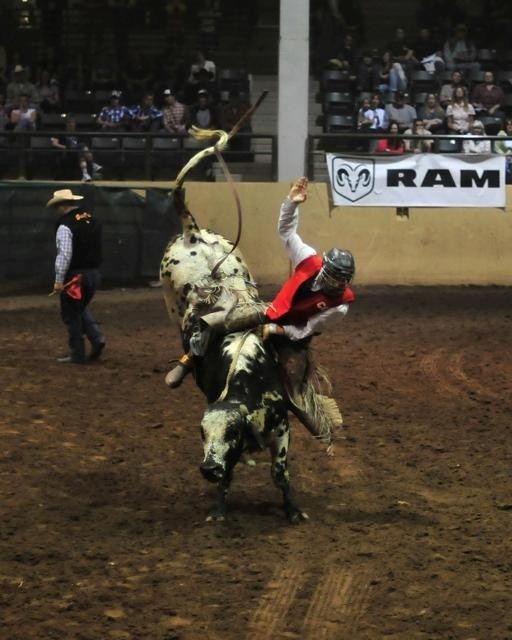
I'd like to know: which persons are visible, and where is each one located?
[164,176,356,444]
[330,1,512,154]
[0,2,252,182]
[46,187,107,363]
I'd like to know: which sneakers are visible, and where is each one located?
[55,341,107,364]
[81,163,103,182]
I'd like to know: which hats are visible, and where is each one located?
[46,188,84,209]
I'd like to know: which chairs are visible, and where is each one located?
[316,51,511,161]
[1,51,221,178]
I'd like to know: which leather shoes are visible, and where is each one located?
[164,354,196,388]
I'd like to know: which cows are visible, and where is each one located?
[159,124,302,523]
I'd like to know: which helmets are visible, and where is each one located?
[322,248,355,274]
